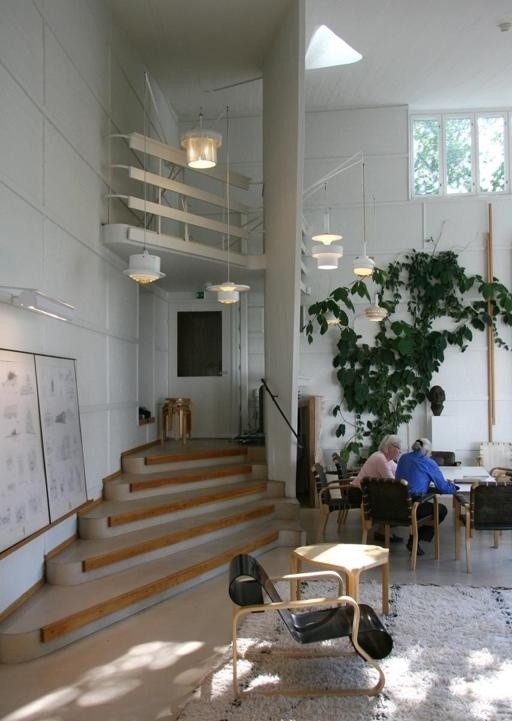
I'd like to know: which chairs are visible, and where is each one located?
[311,463,360,543]
[360,477,440,570]
[476,441,512,474]
[228,553,393,694]
[454,481,512,574]
[431,450,462,466]
[333,453,359,523]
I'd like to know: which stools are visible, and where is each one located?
[289,543,390,619]
[160,396,193,447]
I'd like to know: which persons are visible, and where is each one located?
[394,438,460,555]
[348,434,404,543]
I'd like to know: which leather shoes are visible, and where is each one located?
[374,531,403,543]
[407,541,425,556]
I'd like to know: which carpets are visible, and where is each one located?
[173,574,510,721]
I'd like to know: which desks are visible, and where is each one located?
[344,465,497,555]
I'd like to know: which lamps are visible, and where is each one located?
[321,283,340,325]
[14,287,76,324]
[363,272,388,323]
[178,106,225,170]
[205,105,251,306]
[311,183,345,271]
[352,154,376,276]
[120,73,167,287]
